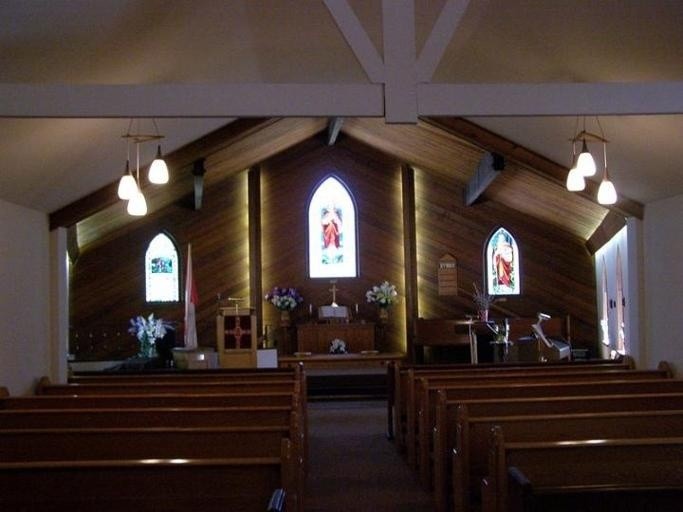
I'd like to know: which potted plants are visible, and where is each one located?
[470,282,494,322]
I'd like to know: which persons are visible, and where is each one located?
[320,197,343,249]
[491,234,513,283]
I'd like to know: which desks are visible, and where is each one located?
[276,351,407,403]
[414,312,572,366]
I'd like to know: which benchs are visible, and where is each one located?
[385,356,683,512]
[0,360,307,512]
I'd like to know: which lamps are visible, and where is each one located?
[566,115,620,206]
[116,117,171,217]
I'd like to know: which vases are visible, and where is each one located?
[280,308,290,323]
[376,304,390,328]
[138,336,159,358]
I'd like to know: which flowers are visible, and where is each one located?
[330,337,347,354]
[125,312,178,346]
[265,284,303,310]
[366,281,401,307]
